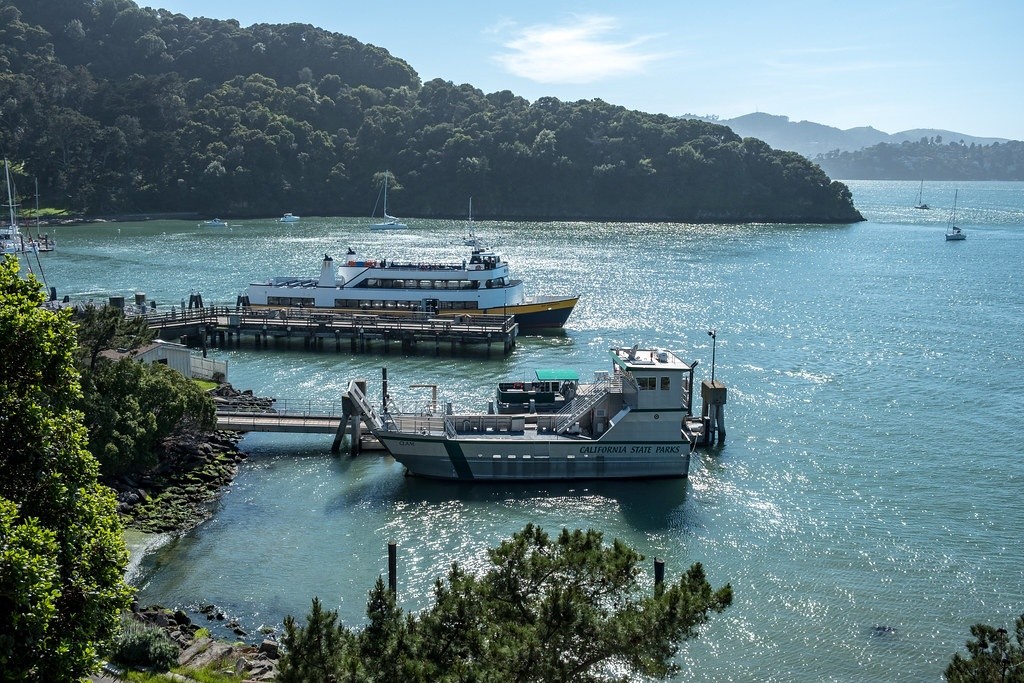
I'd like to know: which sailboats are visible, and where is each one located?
[913,178,929,209]
[945,189,967,240]
[369,170,407,230]
[26,177,57,251]
[0,158,30,254]
[464,197,484,246]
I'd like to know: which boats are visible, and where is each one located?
[204,218,228,226]
[279,213,299,222]
[347,344,700,479]
[248,231,582,328]
[493,369,613,414]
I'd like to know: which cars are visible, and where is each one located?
[32,218,107,224]
